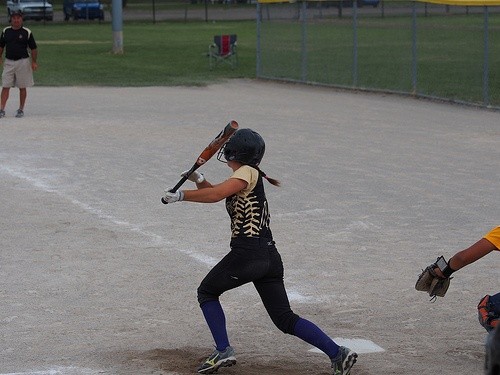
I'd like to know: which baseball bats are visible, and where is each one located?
[160,119,239,205]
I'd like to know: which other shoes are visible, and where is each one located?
[0,110,5,117]
[15,110,23,117]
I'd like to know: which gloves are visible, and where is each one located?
[163,187,184,203]
[180,170,205,184]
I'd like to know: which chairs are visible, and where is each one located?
[209,34,237,72]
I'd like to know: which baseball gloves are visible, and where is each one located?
[414,255,454,303]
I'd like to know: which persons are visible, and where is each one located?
[0,12,39,117]
[415,225,500,375]
[164,127,359,375]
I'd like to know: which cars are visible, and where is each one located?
[62,0,105,22]
[6,0,54,22]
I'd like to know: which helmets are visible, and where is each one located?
[224,128,265,166]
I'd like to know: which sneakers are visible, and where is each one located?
[330,346,359,375]
[197,344,237,373]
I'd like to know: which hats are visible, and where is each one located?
[10,9,22,17]
